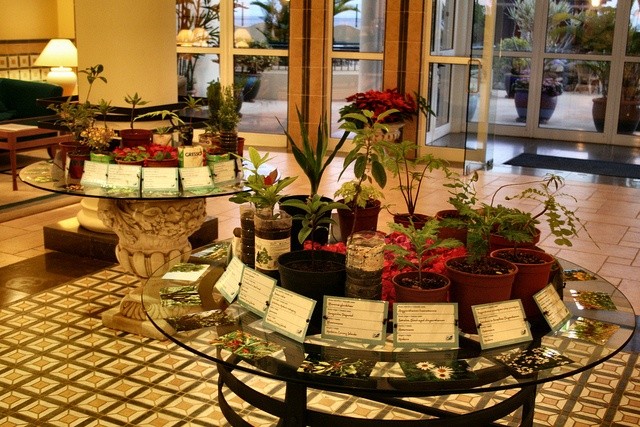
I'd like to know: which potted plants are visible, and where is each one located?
[553,2,640,135]
[175,1,219,94]
[180,94,202,146]
[276,192,352,335]
[227,143,279,264]
[233,42,281,102]
[490,168,603,322]
[439,195,533,336]
[373,136,450,233]
[132,108,204,168]
[380,217,466,304]
[207,79,245,165]
[216,87,239,175]
[435,164,479,248]
[507,0,578,125]
[244,168,300,275]
[115,91,154,150]
[498,37,531,97]
[482,202,542,255]
[152,125,172,147]
[45,63,108,171]
[198,121,220,145]
[206,145,230,175]
[80,124,119,165]
[274,103,351,258]
[46,99,97,180]
[331,104,400,242]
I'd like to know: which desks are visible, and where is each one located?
[140,234,637,425]
[1,122,77,190]
[17,157,282,342]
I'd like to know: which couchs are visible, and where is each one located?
[0,75,64,162]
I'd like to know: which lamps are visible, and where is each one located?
[30,37,78,96]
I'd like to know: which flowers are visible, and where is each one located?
[114,146,148,160]
[415,360,455,381]
[344,86,417,126]
[143,143,179,158]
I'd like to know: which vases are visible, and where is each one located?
[115,158,144,165]
[343,231,388,300]
[362,123,404,159]
[143,157,178,166]
